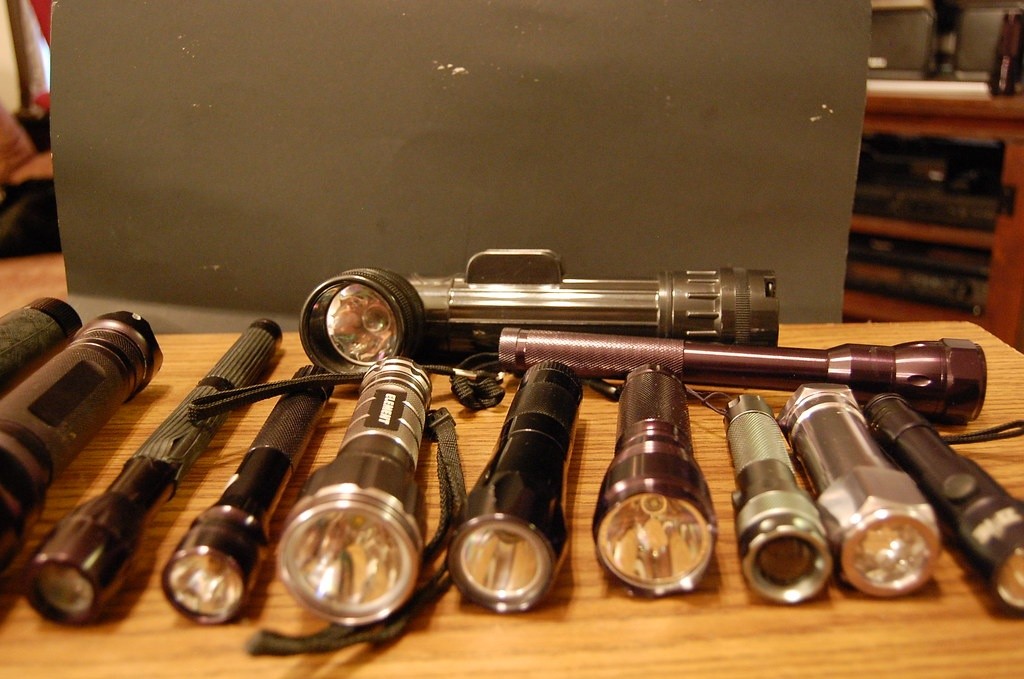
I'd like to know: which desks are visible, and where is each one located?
[0,322,1024,679]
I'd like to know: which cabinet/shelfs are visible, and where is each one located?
[842,81,1024,357]
